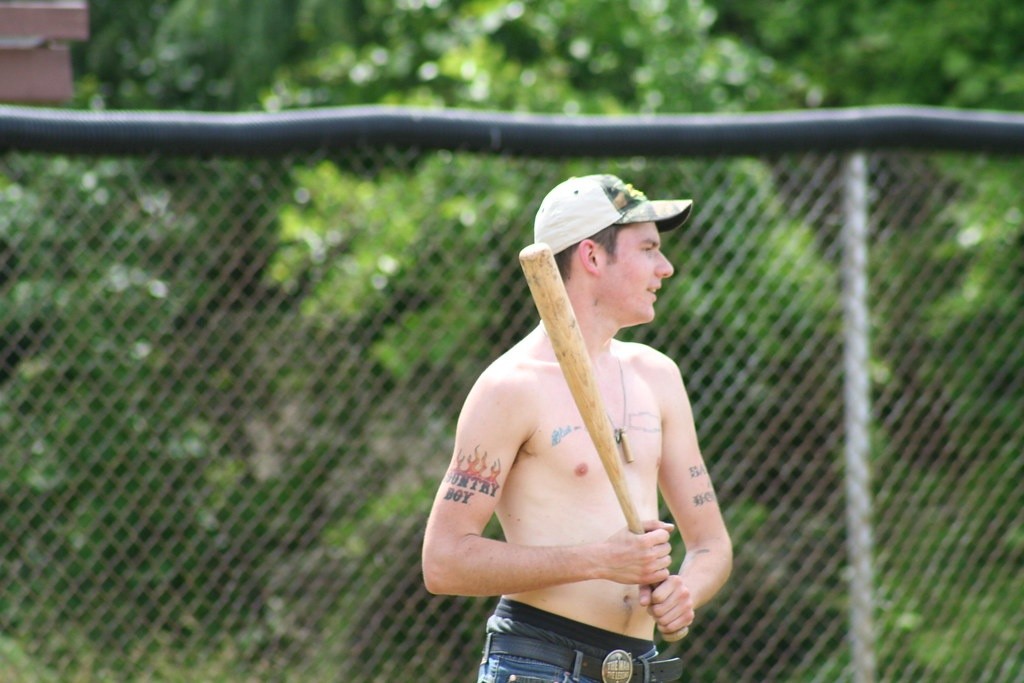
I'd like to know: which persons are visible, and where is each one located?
[423,173,732,683]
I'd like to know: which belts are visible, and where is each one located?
[484,632,684,682]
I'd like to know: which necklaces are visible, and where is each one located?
[606,349,634,463]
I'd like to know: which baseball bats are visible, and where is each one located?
[519,241,689,642]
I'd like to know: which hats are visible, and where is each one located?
[534,172,694,255]
